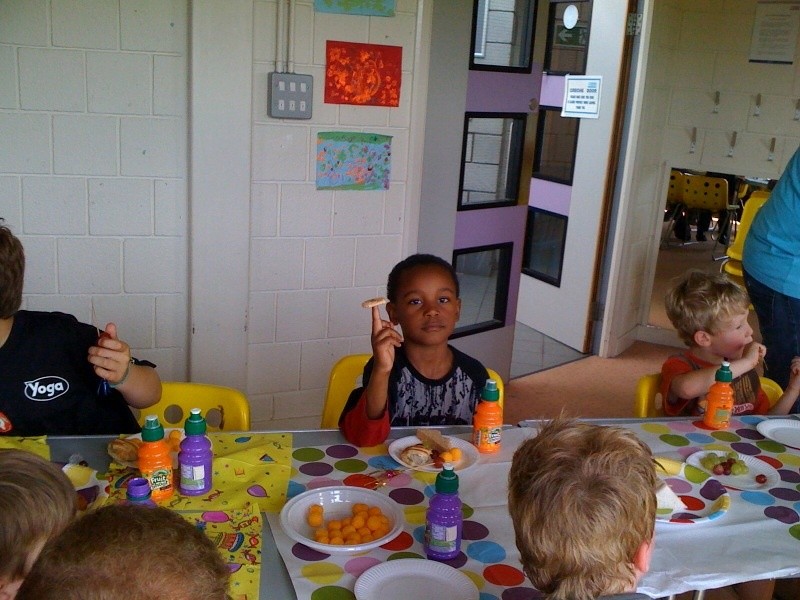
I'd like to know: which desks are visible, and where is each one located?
[731,177,774,219]
[0,414,800,600]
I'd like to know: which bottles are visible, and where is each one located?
[423,463,462,560]
[473,379,503,453]
[703,361,734,428]
[123,408,213,508]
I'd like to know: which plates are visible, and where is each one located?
[388,435,481,473]
[652,458,730,524]
[756,419,800,449]
[686,451,781,491]
[107,429,214,469]
[278,486,405,555]
[49,461,110,521]
[354,558,480,600]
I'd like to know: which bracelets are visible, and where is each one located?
[109,363,130,388]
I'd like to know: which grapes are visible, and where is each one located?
[430,449,445,470]
[701,450,767,483]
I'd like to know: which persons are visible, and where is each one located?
[506,403,668,600]
[0,225,162,476]
[15,501,231,600]
[336,251,491,447]
[659,269,800,416]
[0,446,79,600]
[739,144,800,415]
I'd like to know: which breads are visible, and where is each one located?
[417,428,450,451]
[400,443,434,468]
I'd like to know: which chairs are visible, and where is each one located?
[659,170,773,312]
[630,372,782,419]
[319,355,504,430]
[128,381,248,435]
